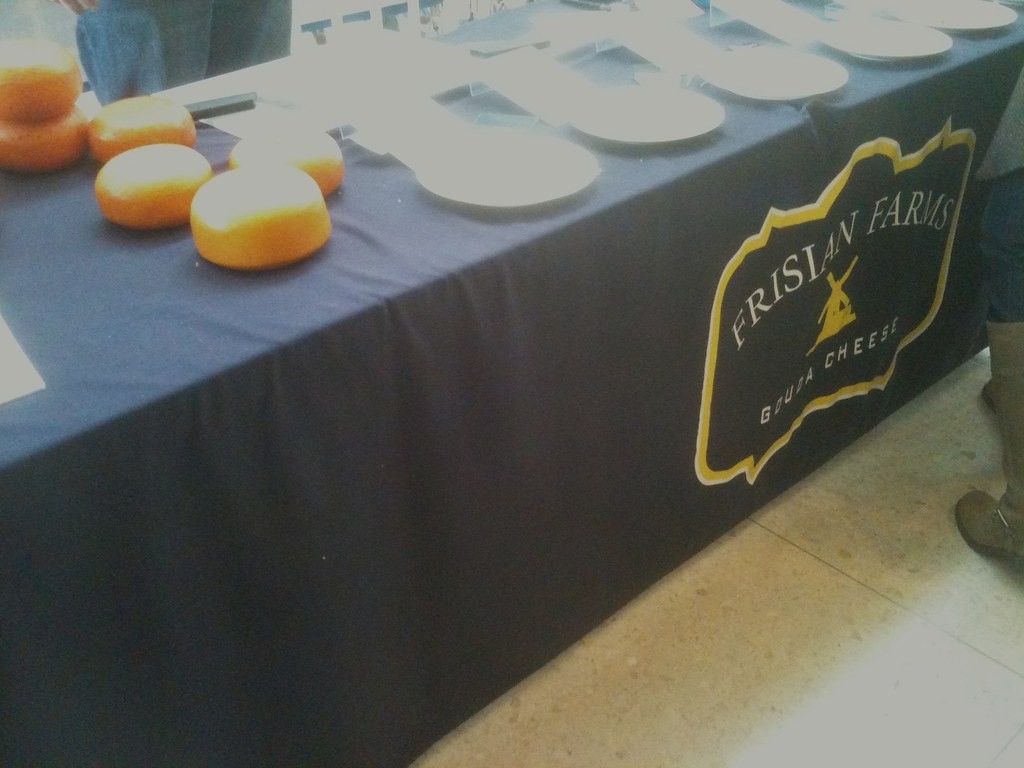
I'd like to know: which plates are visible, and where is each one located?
[701,45,847,102]
[418,129,598,207]
[821,15,955,64]
[887,1,1017,32]
[575,84,724,145]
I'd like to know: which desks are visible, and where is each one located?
[0,0,1024,768]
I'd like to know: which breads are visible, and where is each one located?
[0,38,346,268]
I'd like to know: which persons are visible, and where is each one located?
[54,0,293,107]
[956,63,1024,575]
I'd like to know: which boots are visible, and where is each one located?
[955,320,1024,560]
[982,379,996,408]
